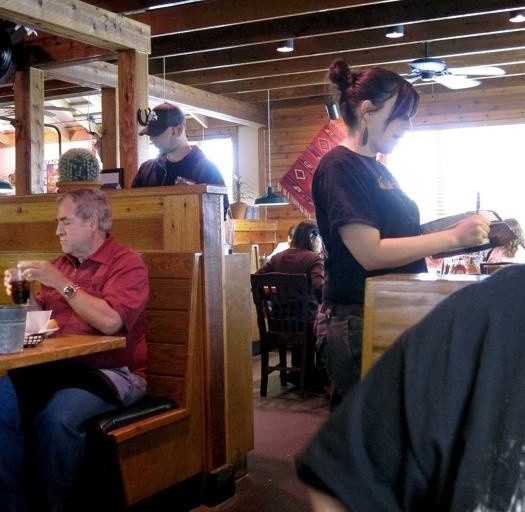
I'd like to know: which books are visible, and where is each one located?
[421,210,518,260]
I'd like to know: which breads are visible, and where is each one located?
[48,319,57,329]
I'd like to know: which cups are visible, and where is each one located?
[0,304,29,355]
[6,261,35,307]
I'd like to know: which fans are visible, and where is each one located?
[398,42,506,89]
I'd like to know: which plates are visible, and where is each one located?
[24,327,59,338]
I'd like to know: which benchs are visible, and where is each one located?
[26,247,207,512]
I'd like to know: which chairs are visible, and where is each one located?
[248,244,320,397]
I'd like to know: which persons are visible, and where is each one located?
[488,216,525,269]
[264,225,296,262]
[292,263,525,512]
[309,56,493,399]
[3,187,151,512]
[0,374,28,512]
[255,221,324,393]
[128,102,231,245]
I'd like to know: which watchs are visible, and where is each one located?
[62,284,81,300]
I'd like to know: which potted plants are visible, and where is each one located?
[228,175,254,219]
[55,147,103,195]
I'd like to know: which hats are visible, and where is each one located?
[139,101,183,137]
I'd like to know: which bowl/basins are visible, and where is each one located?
[24,309,53,334]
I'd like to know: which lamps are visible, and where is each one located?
[275,40,294,52]
[384,25,405,39]
[325,96,339,121]
[509,9,525,23]
[252,89,291,205]
[0,180,13,192]
